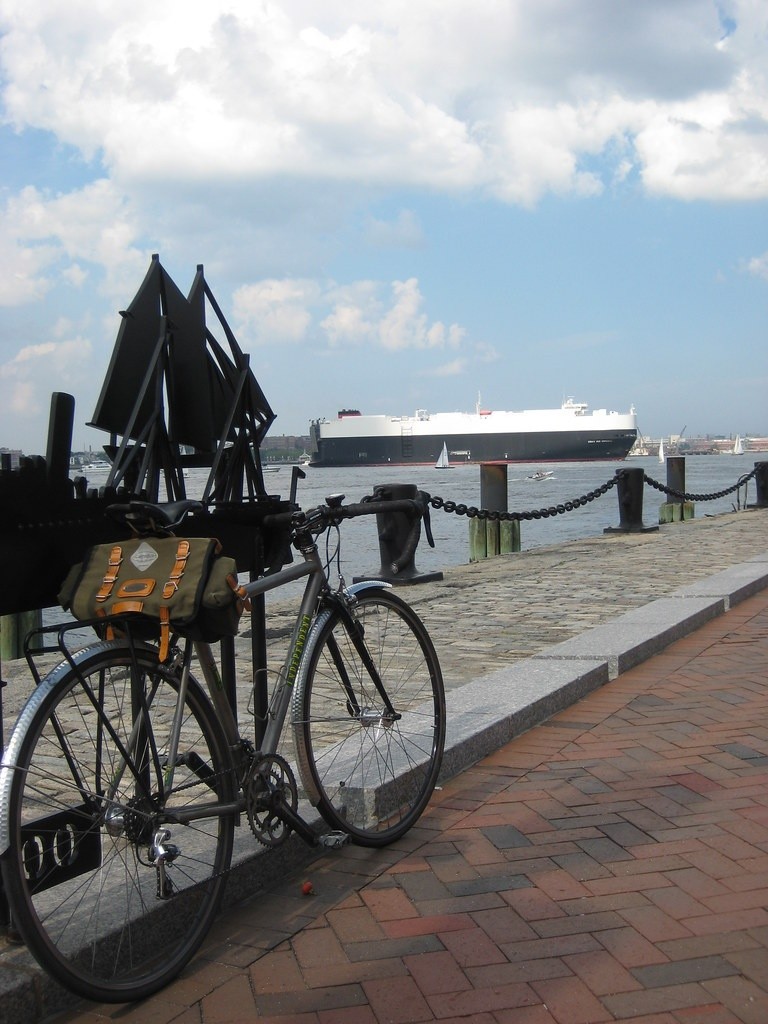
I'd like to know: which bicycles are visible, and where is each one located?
[0,476,447,1006]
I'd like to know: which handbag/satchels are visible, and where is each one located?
[58,537,251,662]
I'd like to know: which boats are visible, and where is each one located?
[308,384,639,462]
[636,425,721,455]
[302,461,310,465]
[261,465,281,472]
[78,460,112,473]
[527,470,555,481]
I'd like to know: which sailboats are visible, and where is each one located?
[162,443,191,478]
[657,436,664,464]
[434,440,454,469]
[729,434,745,455]
